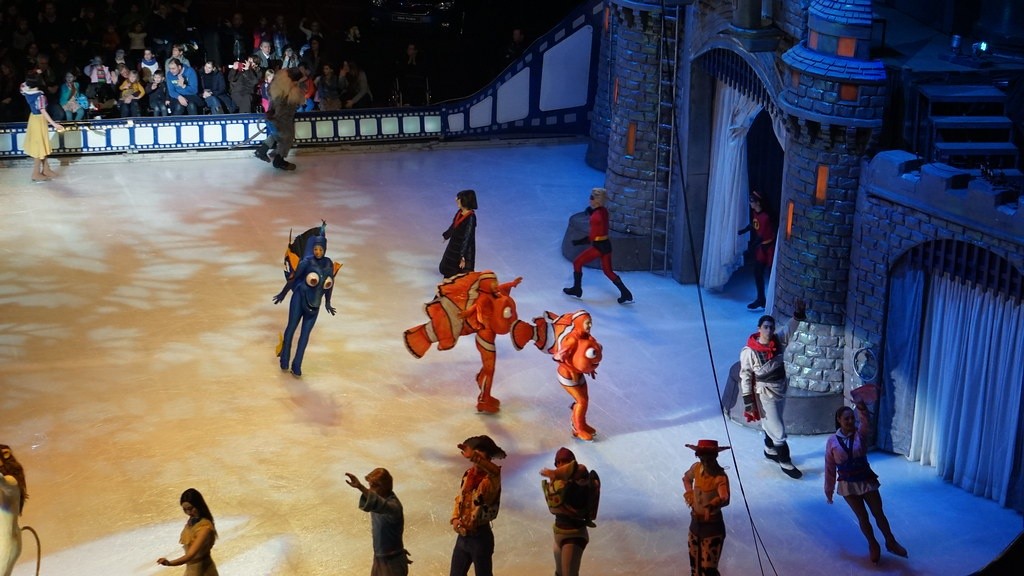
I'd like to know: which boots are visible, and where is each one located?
[612,275,633,303]
[562,271,583,297]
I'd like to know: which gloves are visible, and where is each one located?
[572,236,591,247]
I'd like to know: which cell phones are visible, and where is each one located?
[238,63,244,67]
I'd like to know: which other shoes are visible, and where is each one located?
[778,460,802,479]
[869,541,881,566]
[884,541,908,558]
[273,160,296,170]
[747,297,767,308]
[478,396,500,414]
[571,423,597,441]
[764,450,780,463]
[31,174,49,181]
[254,150,271,163]
[42,169,60,178]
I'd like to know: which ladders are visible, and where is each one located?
[650,5,679,277]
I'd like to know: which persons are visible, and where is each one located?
[540,448,589,576]
[557,309,603,442]
[272,235,337,376]
[563,187,634,304]
[450,435,507,576]
[0,0,431,123]
[737,188,779,308]
[501,28,531,71]
[683,440,732,576]
[345,468,413,576]
[738,297,809,479]
[438,190,478,280]
[156,488,218,576]
[0,444,40,576]
[254,58,306,173]
[457,271,524,414]
[825,396,908,565]
[21,68,67,181]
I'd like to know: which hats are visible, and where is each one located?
[462,435,507,461]
[554,447,576,464]
[684,440,731,454]
